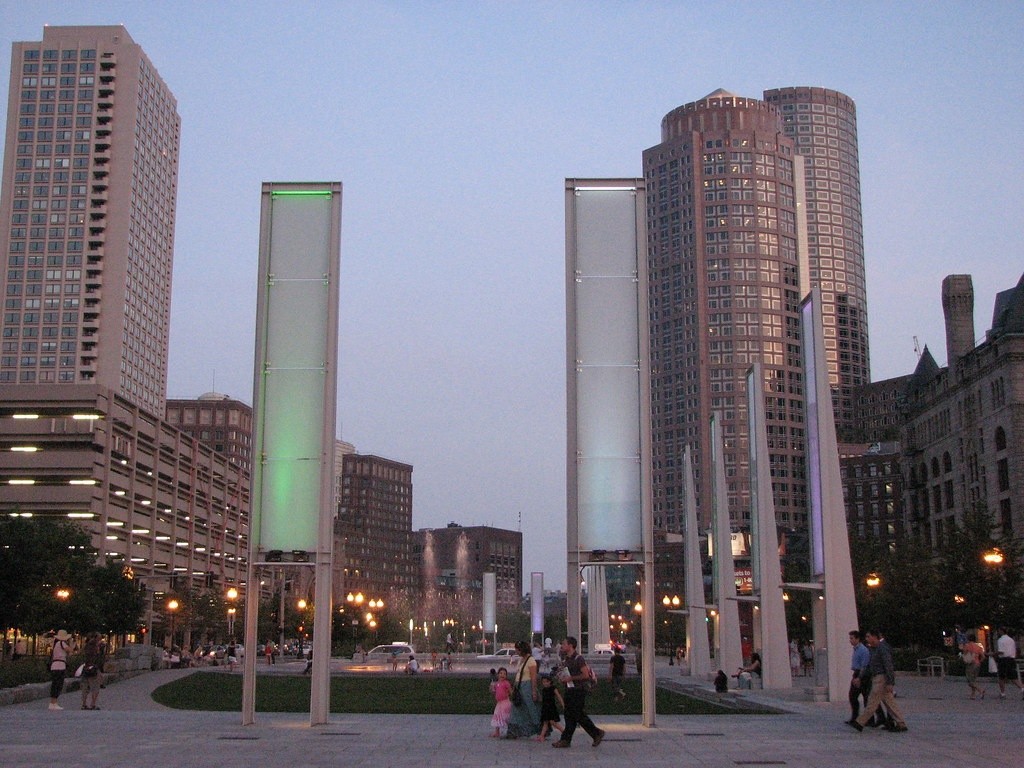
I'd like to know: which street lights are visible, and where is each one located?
[662,594,681,666]
[346,591,364,662]
[167,599,178,654]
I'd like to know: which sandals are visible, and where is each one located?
[81,706,88,709]
[89,705,99,710]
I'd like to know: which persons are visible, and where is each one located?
[16,640,24,653]
[635,643,641,673]
[507,641,553,740]
[392,651,397,671]
[532,642,544,672]
[789,635,814,678]
[488,668,513,738]
[713,670,728,693]
[607,648,626,701]
[265,641,284,665]
[75,632,106,710]
[552,637,605,748]
[48,629,76,710]
[408,656,417,672]
[545,636,553,658]
[354,642,360,654]
[962,634,985,699]
[844,628,908,733]
[731,653,761,679]
[669,647,681,666]
[158,641,237,673]
[447,652,451,671]
[6,640,11,654]
[302,645,313,675]
[990,626,1024,699]
[534,676,565,743]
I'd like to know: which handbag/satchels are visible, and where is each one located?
[988,654,998,674]
[75,664,85,677]
[85,665,97,676]
[512,688,521,706]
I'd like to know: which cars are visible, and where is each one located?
[163,640,312,669]
[476,647,520,662]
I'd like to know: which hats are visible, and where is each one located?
[54,630,71,640]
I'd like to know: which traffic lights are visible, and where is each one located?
[705,617,709,622]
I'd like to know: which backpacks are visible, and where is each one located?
[575,655,597,696]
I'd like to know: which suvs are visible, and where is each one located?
[367,645,416,664]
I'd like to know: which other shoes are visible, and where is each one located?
[592,730,604,746]
[997,692,1005,699]
[845,719,907,733]
[48,703,63,710]
[980,689,985,699]
[552,740,571,747]
[967,695,975,699]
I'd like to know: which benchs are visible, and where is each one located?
[917,656,945,676]
